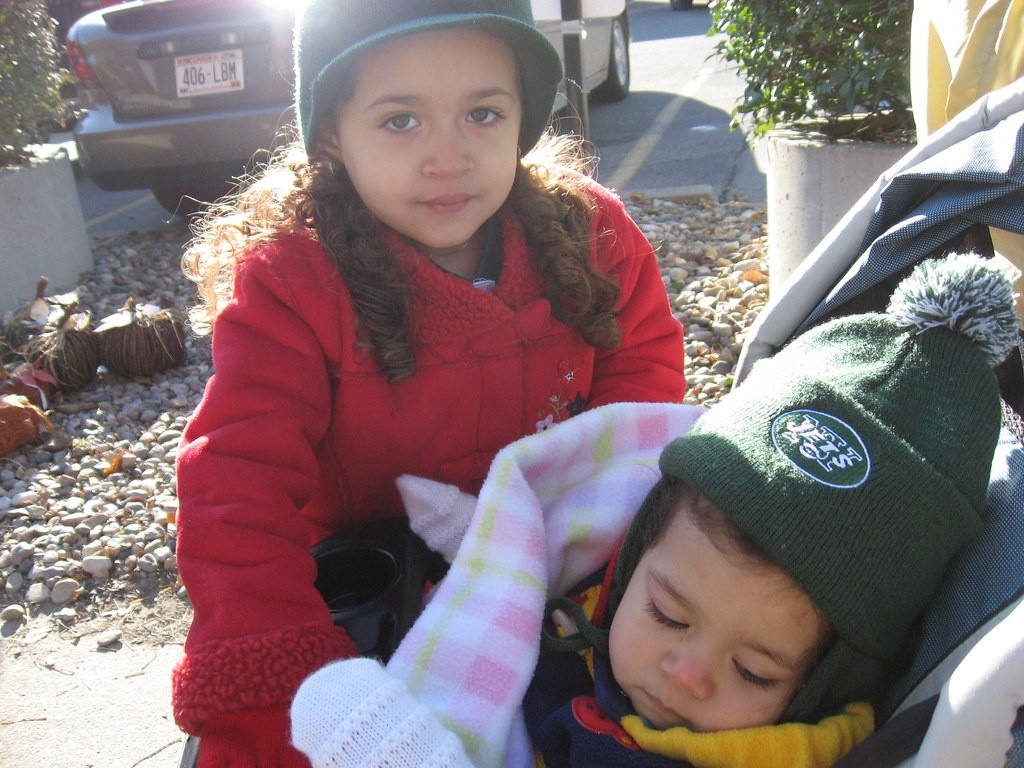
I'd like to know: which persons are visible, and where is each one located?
[173,0,687,768]
[518,252,1018,768]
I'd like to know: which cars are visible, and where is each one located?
[66,1,630,214]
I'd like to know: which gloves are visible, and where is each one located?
[291,657,474,767]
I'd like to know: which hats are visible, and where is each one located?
[294,1,562,162]
[538,249,1020,731]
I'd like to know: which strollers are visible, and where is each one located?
[527,77,1024,768]
